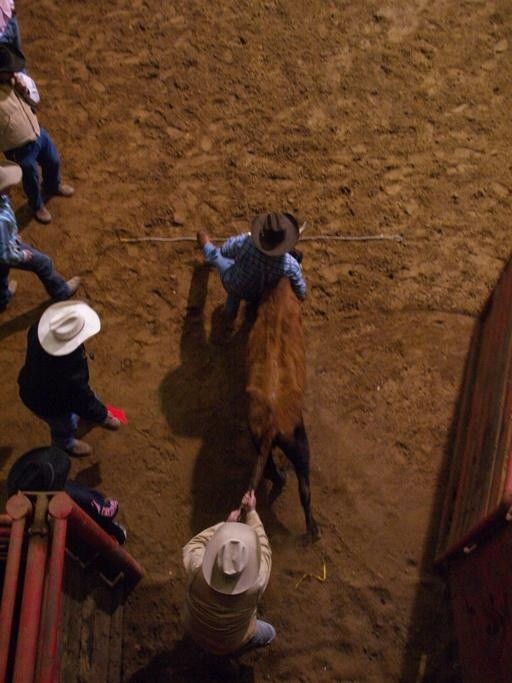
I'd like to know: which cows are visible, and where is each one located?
[239,220,325,541]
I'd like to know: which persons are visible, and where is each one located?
[18,298,121,456]
[178,489,277,674]
[1,164,82,311]
[196,208,308,319]
[3,442,128,544]
[1,0,27,74]
[0,40,74,223]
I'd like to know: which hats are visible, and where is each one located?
[0,159,23,190]
[7,446,71,500]
[0,42,27,71]
[251,211,300,257]
[37,300,101,357]
[202,522,261,596]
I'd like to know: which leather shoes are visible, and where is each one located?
[197,229,209,251]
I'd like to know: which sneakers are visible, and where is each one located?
[7,280,17,296]
[65,276,81,296]
[101,413,120,429]
[51,438,93,457]
[58,180,74,197]
[35,202,52,224]
